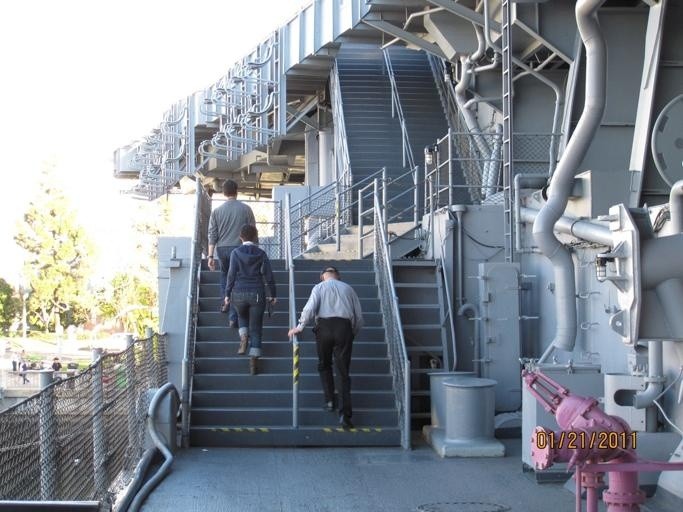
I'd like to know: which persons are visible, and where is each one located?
[206,179,256,313]
[223,223,277,375]
[12,351,63,384]
[287,265,362,428]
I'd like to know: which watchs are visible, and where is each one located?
[206,256,213,259]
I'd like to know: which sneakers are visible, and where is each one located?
[339,414,353,430]
[229,319,238,329]
[220,302,230,313]
[321,400,336,412]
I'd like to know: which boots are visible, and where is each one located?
[249,356,258,376]
[237,334,249,355]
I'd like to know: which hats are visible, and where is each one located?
[320,266,340,274]
